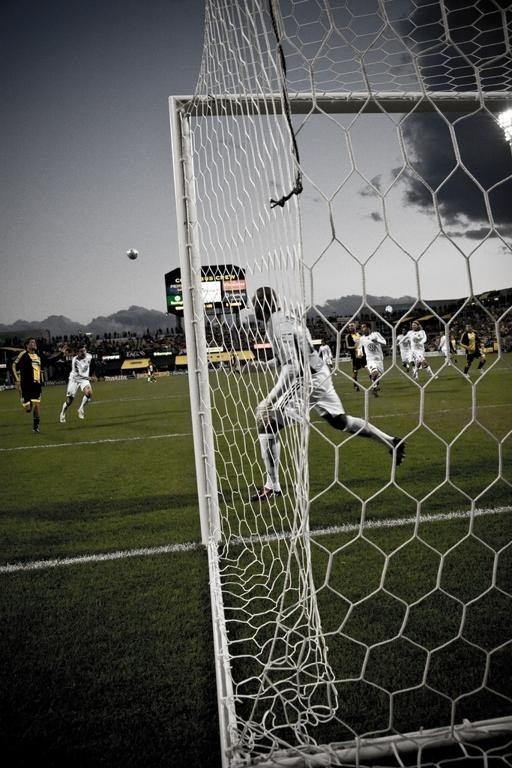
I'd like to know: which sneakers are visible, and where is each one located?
[60,413,66,422]
[78,408,84,419]
[33,428,40,432]
[353,368,484,391]
[390,437,405,466]
[250,486,281,501]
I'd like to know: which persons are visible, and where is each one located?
[60,344,93,424]
[345,322,387,398]
[247,286,406,501]
[391,327,414,371]
[398,320,440,381]
[435,322,459,365]
[459,323,487,379]
[318,340,338,376]
[146,360,160,385]
[12,337,68,433]
[229,350,240,376]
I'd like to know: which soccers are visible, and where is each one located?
[127,248,138,259]
[384,305,392,313]
